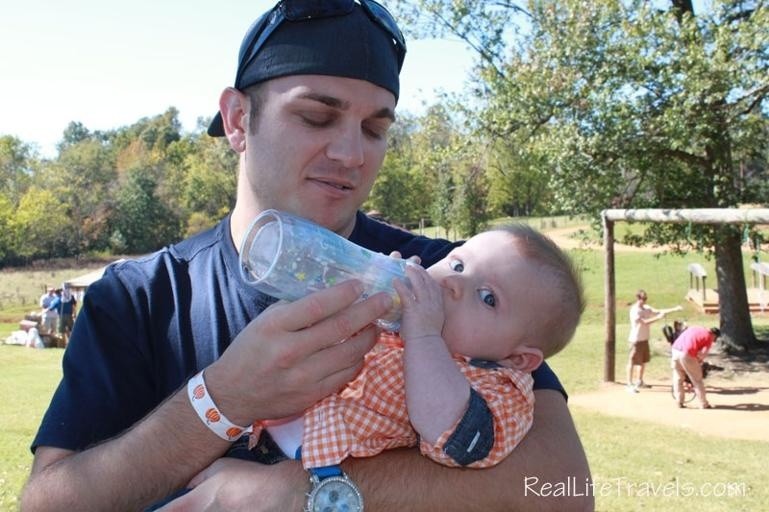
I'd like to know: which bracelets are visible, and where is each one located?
[185,370,249,447]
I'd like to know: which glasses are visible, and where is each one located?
[236,1,407,86]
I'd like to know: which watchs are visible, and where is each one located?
[299,460,365,511]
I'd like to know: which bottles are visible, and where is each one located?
[237,207,417,340]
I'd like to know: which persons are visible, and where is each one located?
[17,0,596,512]
[673,319,724,378]
[668,326,722,410]
[626,289,681,392]
[40,282,79,349]
[220,219,589,477]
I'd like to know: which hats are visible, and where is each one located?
[208,8,399,137]
